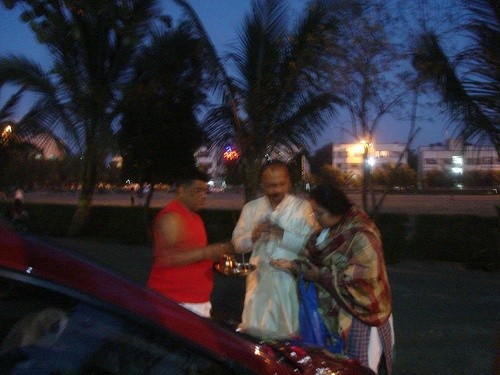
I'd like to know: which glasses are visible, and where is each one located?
[313,208,327,221]
[190,187,212,195]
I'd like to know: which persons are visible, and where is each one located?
[145,168,217,321]
[229,158,322,341]
[271,179,397,375]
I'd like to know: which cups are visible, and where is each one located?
[221,250,235,272]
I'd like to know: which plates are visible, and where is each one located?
[216,263,255,276]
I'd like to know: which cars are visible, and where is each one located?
[0,224,378,375]
